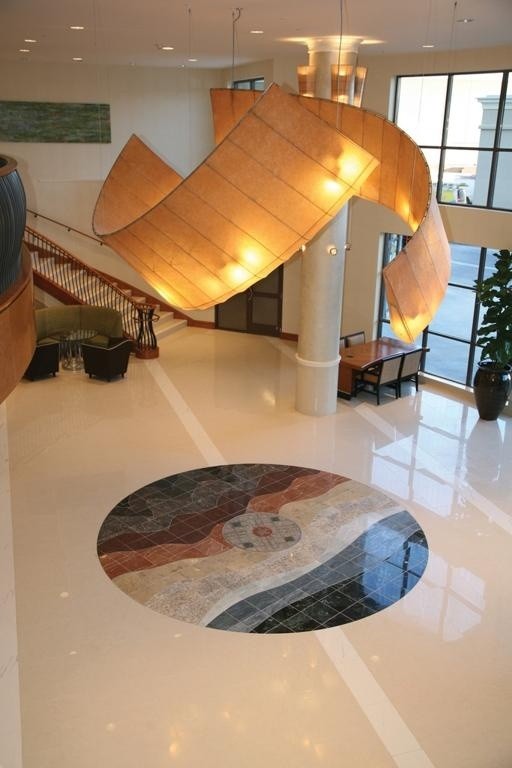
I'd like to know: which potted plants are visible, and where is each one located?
[472,249,512,420]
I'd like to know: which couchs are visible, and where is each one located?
[33,304,122,356]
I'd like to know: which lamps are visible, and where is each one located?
[89,5,453,346]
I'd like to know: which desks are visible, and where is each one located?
[337,337,429,401]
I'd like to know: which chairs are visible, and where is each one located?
[337,331,423,405]
[78,338,132,382]
[23,343,59,381]
